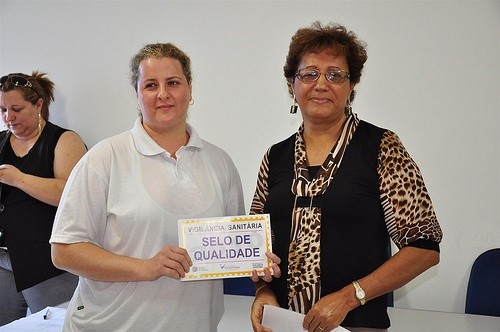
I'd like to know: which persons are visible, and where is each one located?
[48,43,281,332]
[0,70,89,327]
[249,20,443,332]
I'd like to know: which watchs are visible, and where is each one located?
[351,281,367,306]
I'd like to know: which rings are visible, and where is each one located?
[318,325,324,331]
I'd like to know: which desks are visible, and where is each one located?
[0,293,500,332]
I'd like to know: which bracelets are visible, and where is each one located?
[255,284,272,297]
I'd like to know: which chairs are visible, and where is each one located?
[465,247,500,317]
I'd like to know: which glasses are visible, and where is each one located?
[0,76,41,98]
[295,67,351,83]
